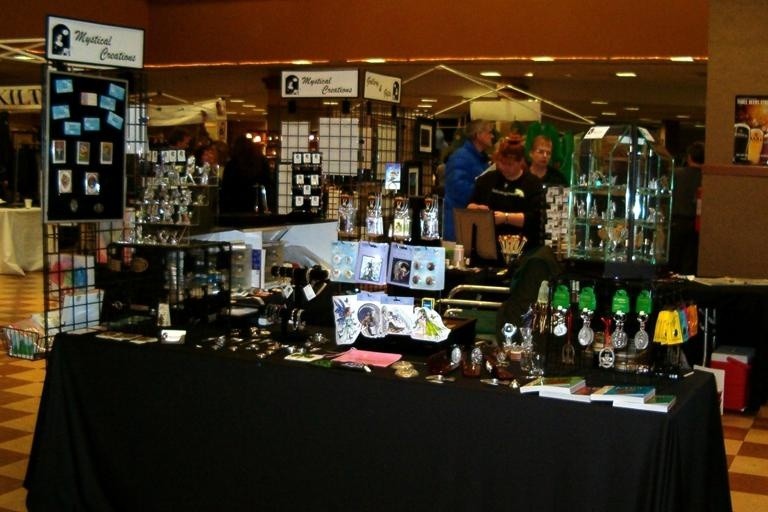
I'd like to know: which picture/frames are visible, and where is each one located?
[412,118,437,157]
[732,91,768,173]
[403,160,423,199]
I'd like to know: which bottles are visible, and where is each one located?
[450,244,465,268]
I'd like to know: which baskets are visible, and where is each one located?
[0,327,57,361]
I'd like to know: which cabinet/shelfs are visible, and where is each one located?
[126,169,225,250]
[438,252,767,416]
[107,236,236,333]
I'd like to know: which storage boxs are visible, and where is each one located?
[707,343,753,416]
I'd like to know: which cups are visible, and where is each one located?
[734,124,750,160]
[748,129,763,163]
[24,199,31,209]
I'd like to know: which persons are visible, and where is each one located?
[464,132,546,258]
[523,135,568,188]
[149,123,274,213]
[435,158,450,187]
[666,142,706,274]
[442,119,495,243]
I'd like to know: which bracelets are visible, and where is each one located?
[261,262,319,332]
[504,212,508,225]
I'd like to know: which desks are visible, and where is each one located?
[0,206,60,276]
[23,309,731,509]
